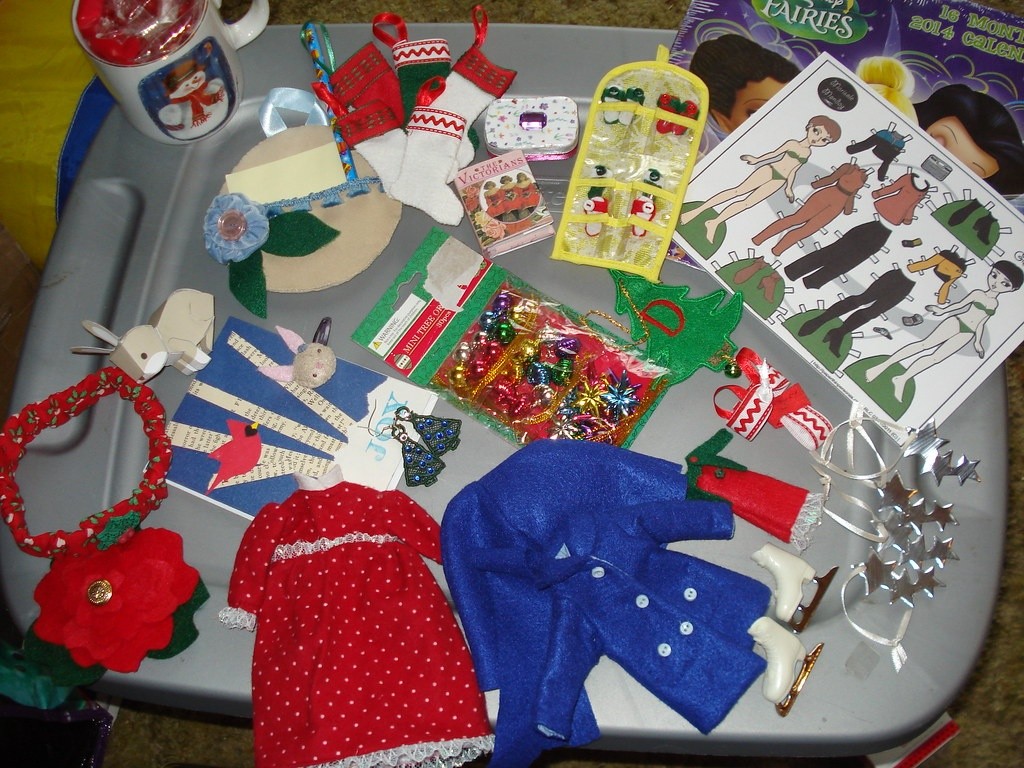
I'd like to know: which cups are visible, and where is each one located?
[69,1,271,147]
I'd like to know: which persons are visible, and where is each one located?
[483,172,540,222]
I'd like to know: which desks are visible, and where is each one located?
[0,25,1009,758]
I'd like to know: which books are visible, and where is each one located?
[453,150,556,259]
[144,315,440,519]
[665,0,1024,271]
